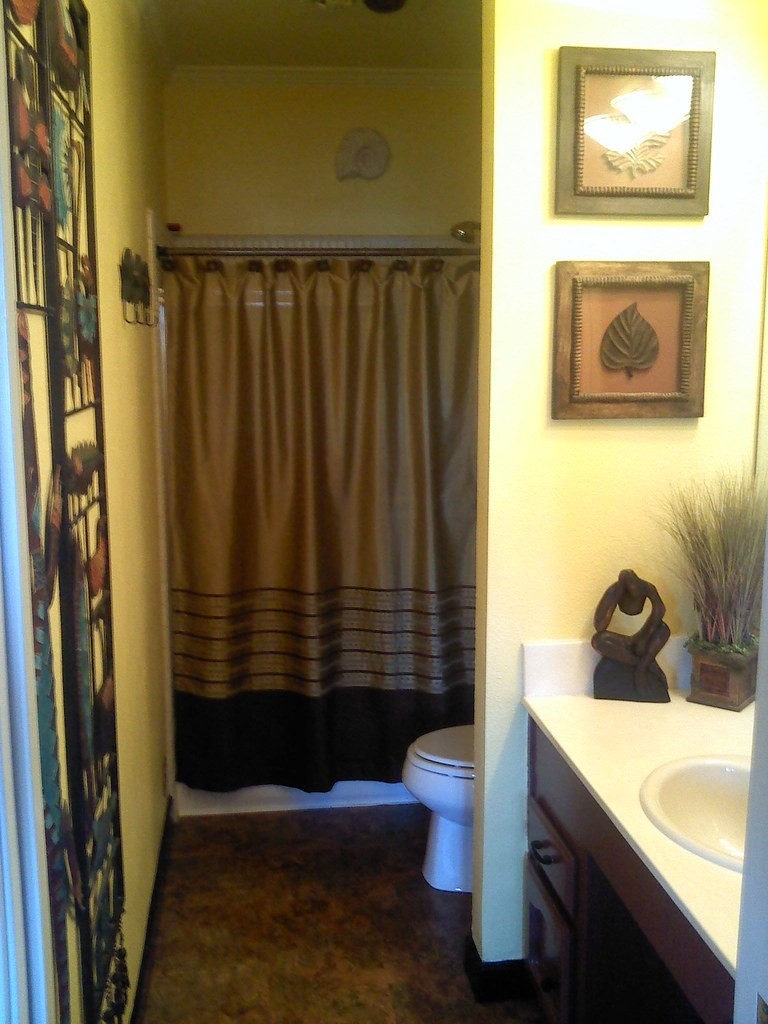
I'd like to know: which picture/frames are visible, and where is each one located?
[557,45,718,219]
[552,260,712,420]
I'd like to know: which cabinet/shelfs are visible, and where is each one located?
[523,719,735,1024]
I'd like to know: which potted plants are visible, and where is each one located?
[649,480,768,711]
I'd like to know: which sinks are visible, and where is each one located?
[639,754,752,873]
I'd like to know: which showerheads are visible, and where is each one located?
[448,220,481,243]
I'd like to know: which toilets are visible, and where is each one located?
[400,724,477,893]
